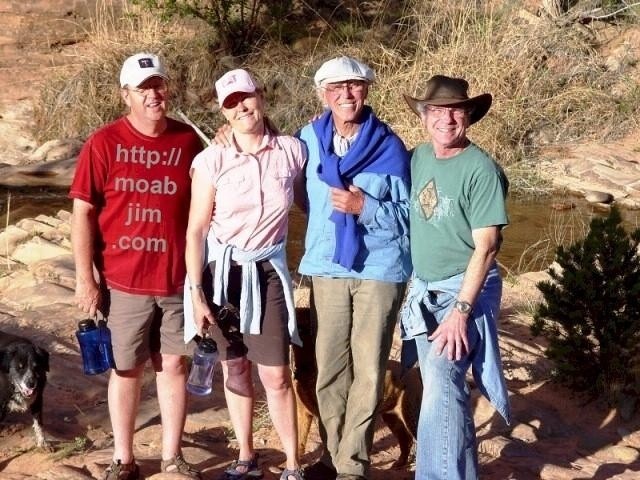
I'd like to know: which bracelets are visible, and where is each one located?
[190,284,203,290]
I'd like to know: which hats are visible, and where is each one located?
[214,68,259,108]
[403,75,493,127]
[314,56,375,87]
[120,53,169,88]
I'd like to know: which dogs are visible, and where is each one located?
[293,306,422,471]
[0,331,56,454]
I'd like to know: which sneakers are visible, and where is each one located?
[220,451,263,479]
[280,468,305,480]
[98,455,139,480]
[161,451,200,480]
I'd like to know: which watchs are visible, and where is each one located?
[453,301,474,316]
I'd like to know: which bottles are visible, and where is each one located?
[185,333,219,396]
[76,319,111,376]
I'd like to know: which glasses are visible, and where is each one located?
[426,106,470,116]
[132,84,168,93]
[325,82,365,92]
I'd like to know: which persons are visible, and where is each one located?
[307,74,510,480]
[183,70,326,479]
[209,57,410,479]
[67,51,205,480]
